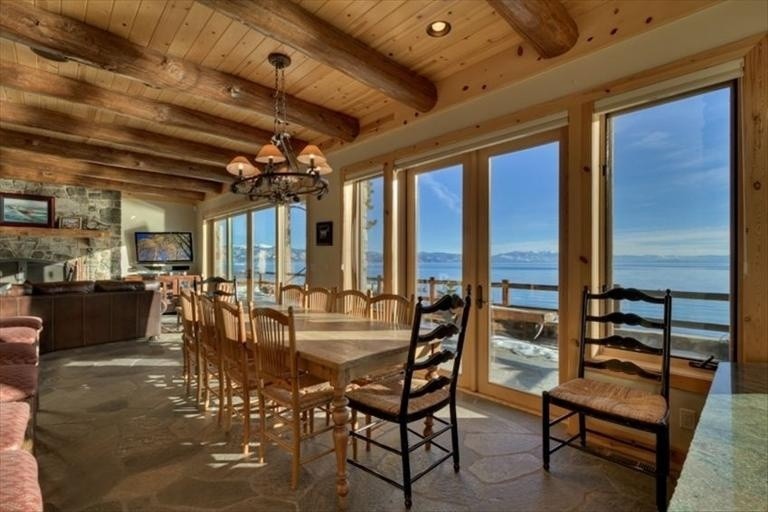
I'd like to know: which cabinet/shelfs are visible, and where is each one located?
[122,274,202,315]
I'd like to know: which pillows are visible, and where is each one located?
[1,281,145,296]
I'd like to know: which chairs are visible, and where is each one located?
[542,284,672,512]
[1,315,49,367]
[179,276,473,507]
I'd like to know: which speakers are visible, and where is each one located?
[144,266,191,271]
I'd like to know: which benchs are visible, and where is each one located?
[490,304,557,341]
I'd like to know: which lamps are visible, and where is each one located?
[226,52,333,206]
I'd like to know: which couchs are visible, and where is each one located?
[0,364,44,512]
[0,280,154,351]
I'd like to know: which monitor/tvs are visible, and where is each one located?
[135,232,193,266]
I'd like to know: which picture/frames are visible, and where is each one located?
[58,216,83,229]
[0,192,55,228]
[316,221,334,246]
